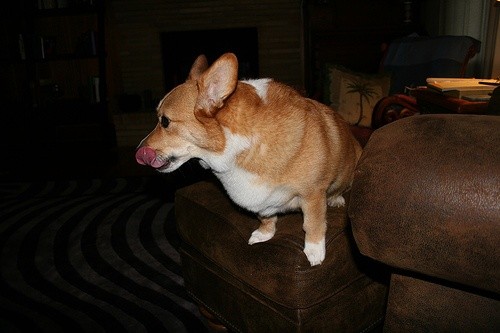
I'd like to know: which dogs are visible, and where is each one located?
[135,52,363,268]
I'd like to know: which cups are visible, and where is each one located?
[88,76,100,103]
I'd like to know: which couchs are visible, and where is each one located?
[347,114,500,333]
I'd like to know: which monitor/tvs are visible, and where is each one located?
[159,26,260,91]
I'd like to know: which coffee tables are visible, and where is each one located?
[372,86,500,130]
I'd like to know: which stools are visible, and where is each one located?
[174,176,392,332]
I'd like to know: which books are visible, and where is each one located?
[426,77,500,102]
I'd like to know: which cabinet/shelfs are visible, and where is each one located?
[111,93,159,175]
[1,2,113,180]
